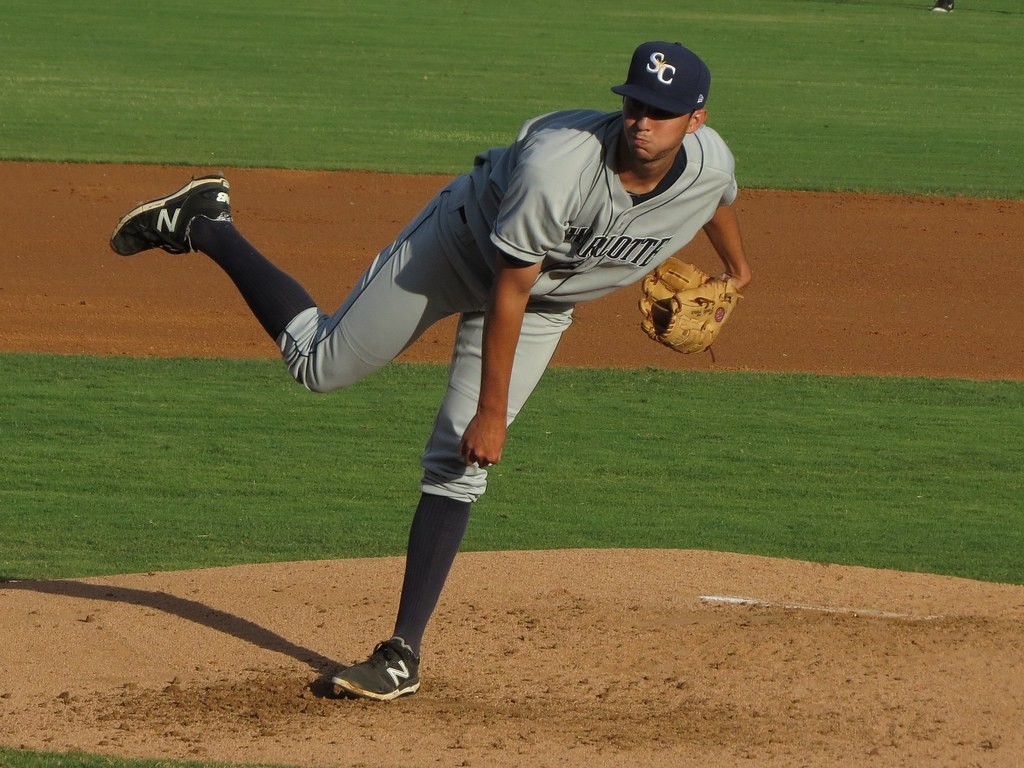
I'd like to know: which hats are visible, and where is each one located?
[610,41,711,113]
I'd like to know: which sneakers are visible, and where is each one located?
[110,175,233,256]
[331,638,421,699]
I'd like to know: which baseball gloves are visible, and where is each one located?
[637,256,740,356]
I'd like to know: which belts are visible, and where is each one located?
[459,205,467,225]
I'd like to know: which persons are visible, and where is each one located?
[106,41,751,701]
